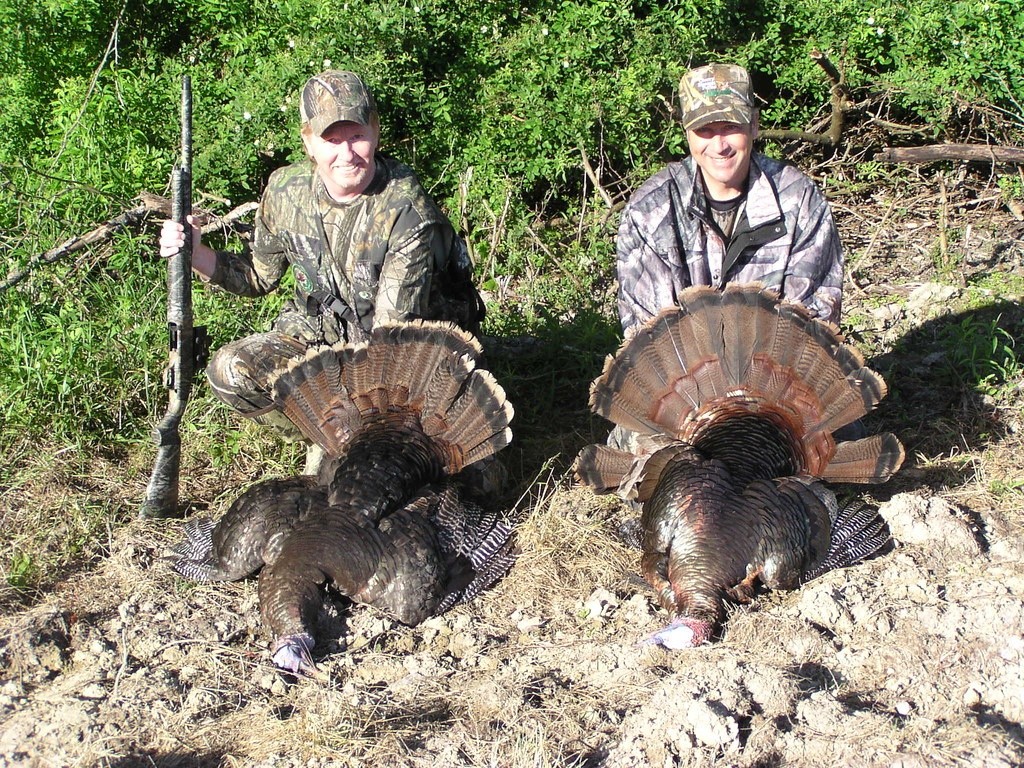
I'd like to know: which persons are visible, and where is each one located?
[603,64,869,458]
[160,69,484,459]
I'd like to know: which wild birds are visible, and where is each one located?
[172,316,522,679]
[564,285,909,653]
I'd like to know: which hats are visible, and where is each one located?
[299,69,370,136]
[678,62,753,131]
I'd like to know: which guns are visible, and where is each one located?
[139,75,195,515]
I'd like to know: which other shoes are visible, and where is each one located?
[301,443,328,477]
